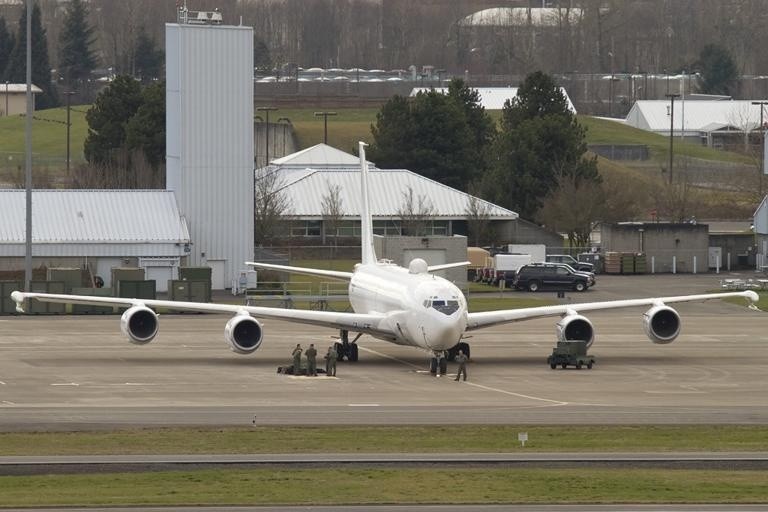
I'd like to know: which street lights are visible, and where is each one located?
[256,107,279,166]
[60,91,76,178]
[663,89,683,185]
[3,78,11,115]
[313,111,337,145]
[751,98,767,193]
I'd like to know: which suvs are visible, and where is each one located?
[475,251,597,293]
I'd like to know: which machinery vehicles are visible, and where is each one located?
[545,341,597,369]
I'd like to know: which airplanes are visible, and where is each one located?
[11,136,759,375]
